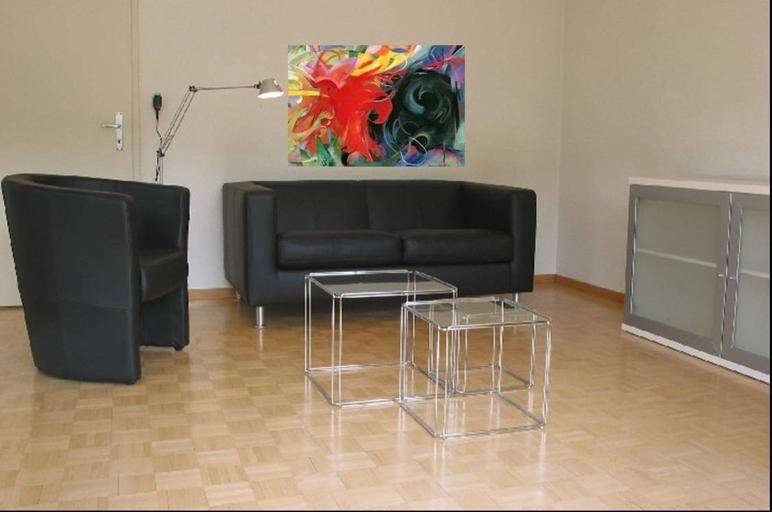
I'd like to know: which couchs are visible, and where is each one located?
[222,181,536,331]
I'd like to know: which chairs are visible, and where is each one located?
[1,175,189,384]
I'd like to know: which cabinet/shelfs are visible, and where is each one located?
[622,175,772,385]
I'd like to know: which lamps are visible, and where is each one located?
[157,78,283,184]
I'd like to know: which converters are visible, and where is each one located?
[153,96,162,111]
